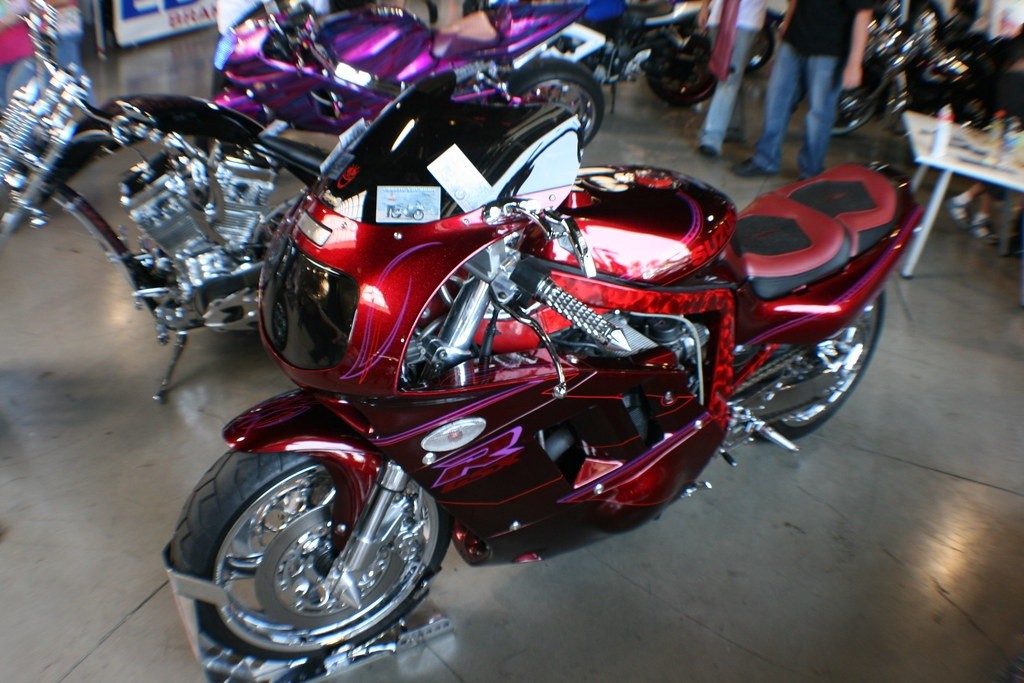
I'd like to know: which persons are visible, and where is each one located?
[694,0,788,160]
[729,0,876,182]
[945,178,1001,245]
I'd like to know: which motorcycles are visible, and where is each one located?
[0,0,1023,683]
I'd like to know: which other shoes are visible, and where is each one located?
[733,158,763,176]
[945,197,970,229]
[699,144,718,158]
[968,221,999,245]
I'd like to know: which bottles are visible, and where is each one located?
[929,104,953,157]
[997,117,1019,172]
[984,110,1007,167]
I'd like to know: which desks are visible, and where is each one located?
[901,109,1023,277]
